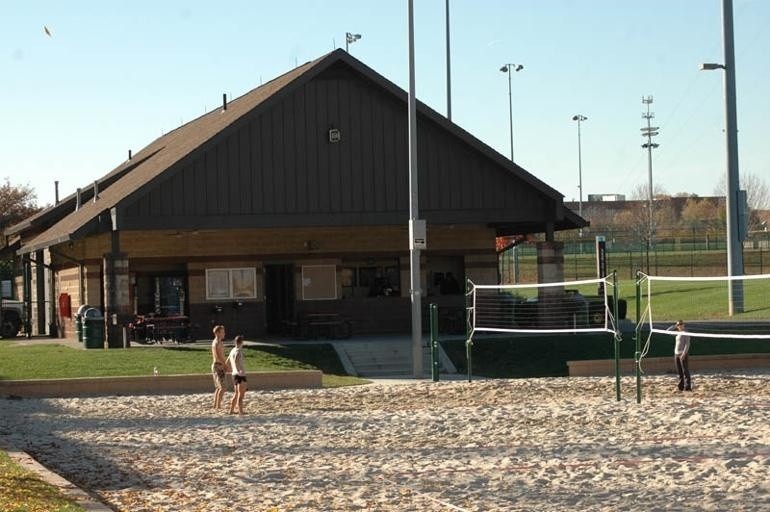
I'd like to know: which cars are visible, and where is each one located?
[1,298,25,338]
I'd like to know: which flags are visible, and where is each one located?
[346,33,361,44]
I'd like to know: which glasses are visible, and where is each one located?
[677,323,684,326]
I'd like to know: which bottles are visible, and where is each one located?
[154,366,158,376]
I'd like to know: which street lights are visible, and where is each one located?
[696,3,747,314]
[638,94,661,232]
[573,113,589,254]
[346,32,362,56]
[500,62,524,162]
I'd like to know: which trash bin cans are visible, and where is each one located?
[74,304,105,348]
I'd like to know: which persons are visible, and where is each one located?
[674,320,691,393]
[210,324,226,411]
[224,336,249,416]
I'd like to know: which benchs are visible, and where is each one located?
[306,313,343,338]
[134,316,188,341]
